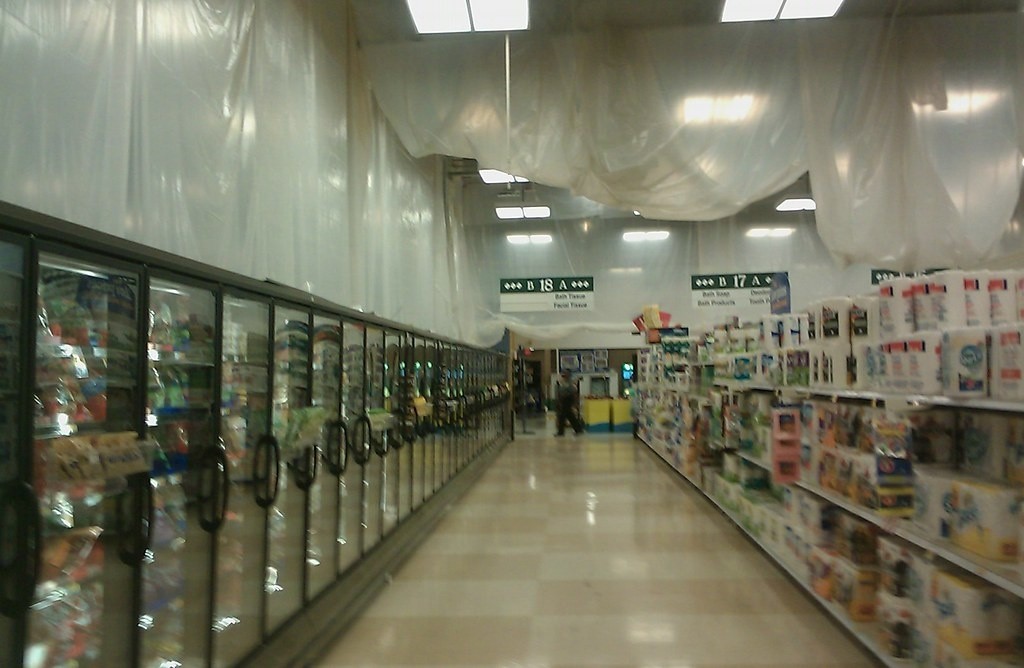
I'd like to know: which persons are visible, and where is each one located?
[553,370,584,437]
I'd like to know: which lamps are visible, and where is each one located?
[720,0,844,23]
[406,0,530,34]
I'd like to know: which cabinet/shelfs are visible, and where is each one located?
[634,377,1024,668]
